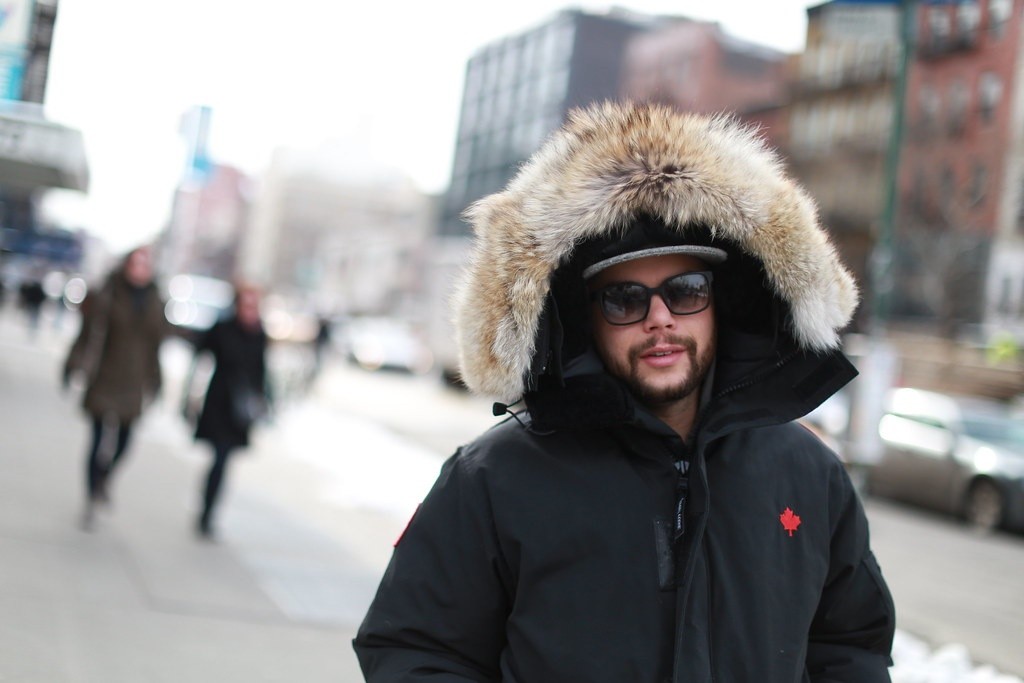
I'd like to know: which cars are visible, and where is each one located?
[871,384,1023,539]
[335,315,419,376]
[165,276,234,349]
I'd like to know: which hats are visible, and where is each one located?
[576,217,729,280]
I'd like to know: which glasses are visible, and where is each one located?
[592,268,715,324]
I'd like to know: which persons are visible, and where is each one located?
[185,285,268,532]
[59,247,197,504]
[352,102,896,683]
